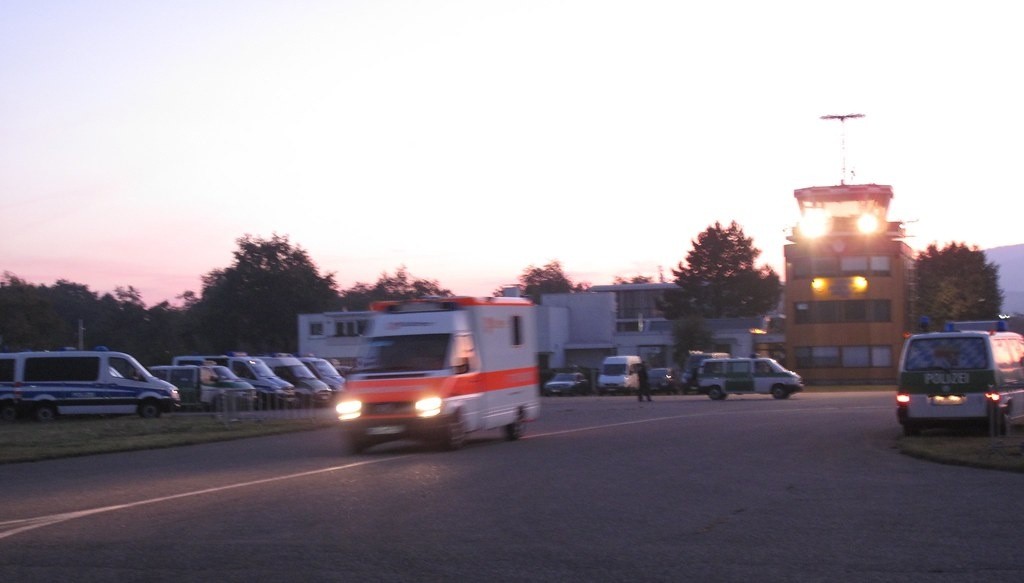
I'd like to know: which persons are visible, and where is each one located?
[639,363,653,402]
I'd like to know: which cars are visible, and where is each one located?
[543,372,589,396]
[647,367,674,395]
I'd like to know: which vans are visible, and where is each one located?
[597,355,642,396]
[679,349,732,394]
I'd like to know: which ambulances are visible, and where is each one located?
[895,315,1023,440]
[1,340,346,424]
[336,291,540,453]
[698,353,805,401]
